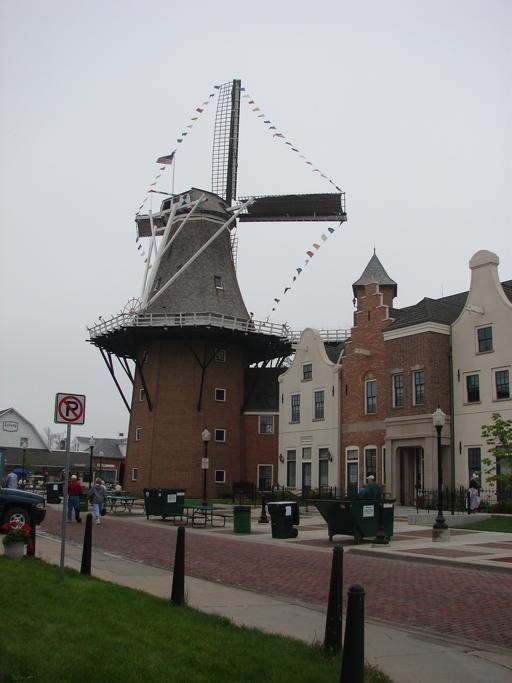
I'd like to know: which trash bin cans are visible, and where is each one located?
[311,498,394,544]
[143,488,186,520]
[268,503,299,539]
[79,494,88,511]
[45,482,64,504]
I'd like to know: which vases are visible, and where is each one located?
[6,541,25,560]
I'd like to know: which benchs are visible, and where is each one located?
[124,503,143,512]
[172,514,205,528]
[103,505,123,513]
[192,512,233,527]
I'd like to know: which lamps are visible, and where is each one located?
[326,451,332,462]
[279,454,284,464]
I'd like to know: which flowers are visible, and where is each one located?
[3,520,35,551]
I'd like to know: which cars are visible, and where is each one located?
[0,487,46,534]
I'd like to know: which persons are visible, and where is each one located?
[362,474,380,499]
[2,469,122,524]
[466,472,483,511]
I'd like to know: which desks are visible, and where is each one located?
[105,496,137,512]
[177,503,225,527]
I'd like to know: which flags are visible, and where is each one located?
[155,154,174,164]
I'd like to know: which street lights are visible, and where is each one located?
[22,441,28,485]
[430,404,448,529]
[88,434,96,490]
[98,448,104,478]
[201,428,210,505]
